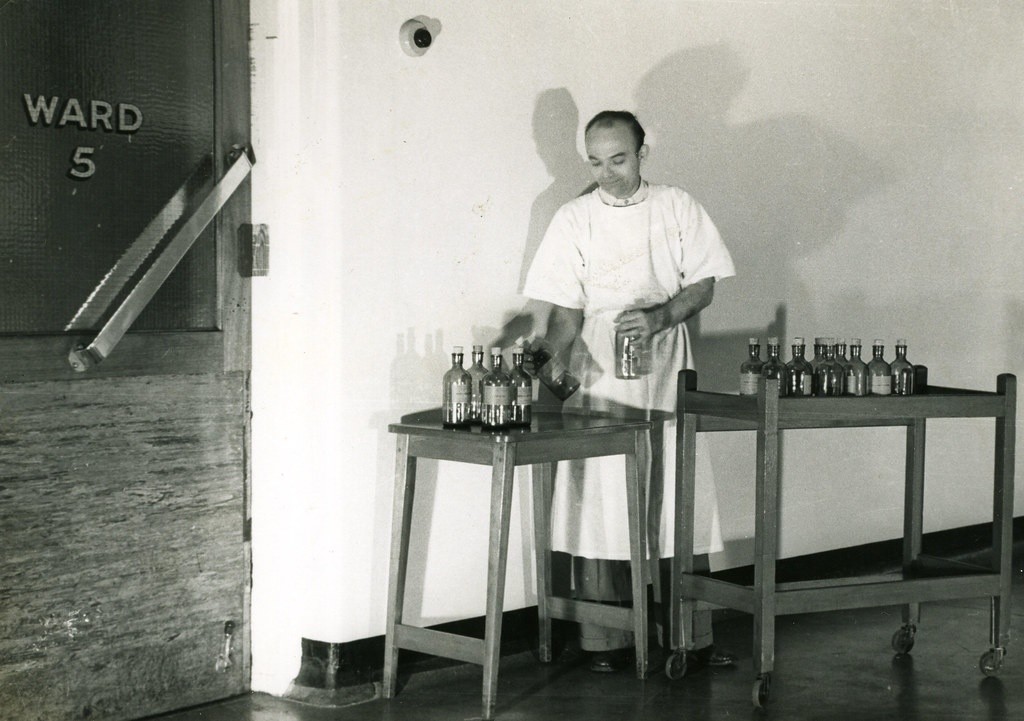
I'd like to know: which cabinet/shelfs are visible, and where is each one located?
[662,366,1017,709]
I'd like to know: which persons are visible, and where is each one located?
[494,107,735,672]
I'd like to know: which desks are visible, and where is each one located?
[382,405,656,721]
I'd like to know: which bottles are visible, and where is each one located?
[866,339,892,396]
[516,337,581,401]
[441,345,471,428]
[467,344,489,423]
[809,338,827,373]
[506,348,532,426]
[613,305,642,379]
[835,337,849,367]
[762,336,786,398]
[889,338,914,396]
[785,338,814,396]
[844,338,868,395]
[481,347,511,433]
[740,336,765,396]
[817,339,843,397]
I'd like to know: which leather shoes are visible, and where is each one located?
[590,644,626,673]
[662,632,737,669]
